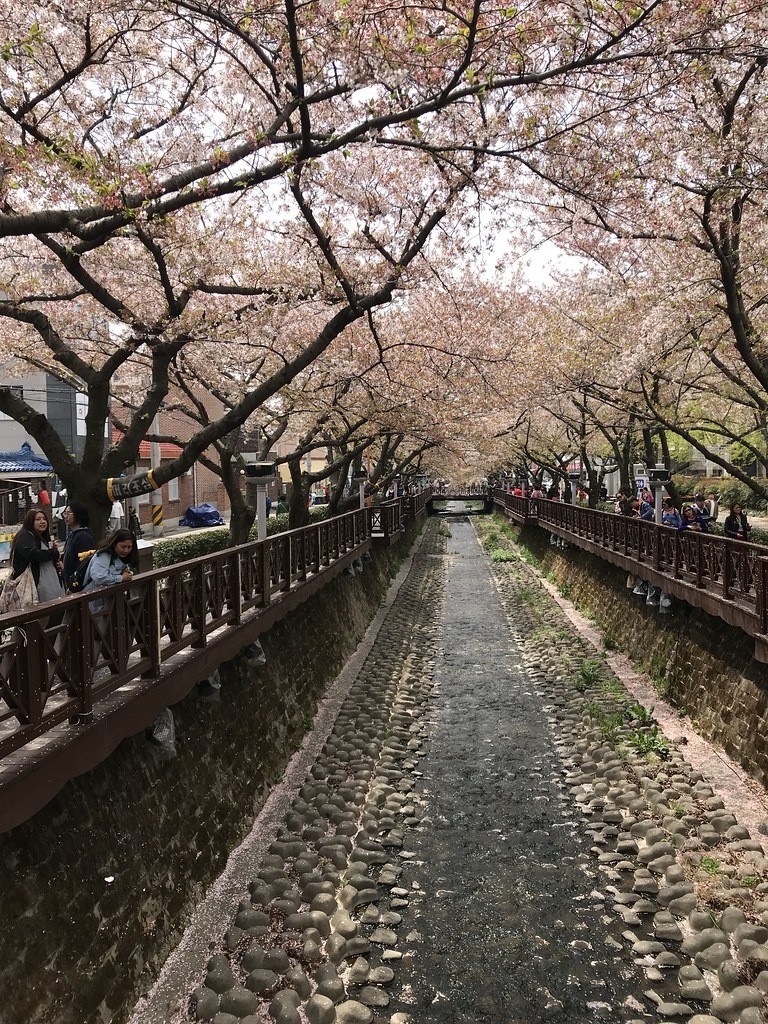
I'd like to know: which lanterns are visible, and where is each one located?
[39,489,50,506]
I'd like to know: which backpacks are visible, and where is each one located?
[67,548,114,593]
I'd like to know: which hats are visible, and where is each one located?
[696,495,706,501]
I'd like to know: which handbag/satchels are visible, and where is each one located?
[0,531,40,613]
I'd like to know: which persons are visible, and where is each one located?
[108,499,125,538]
[266,494,271,518]
[275,493,290,518]
[11,508,67,605]
[704,491,719,523]
[82,528,136,627]
[306,476,657,523]
[724,502,751,552]
[661,498,683,528]
[59,500,98,597]
[128,505,142,539]
[678,504,708,534]
[690,493,711,528]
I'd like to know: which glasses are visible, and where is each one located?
[63,512,74,517]
[685,508,691,512]
[617,496,622,499]
